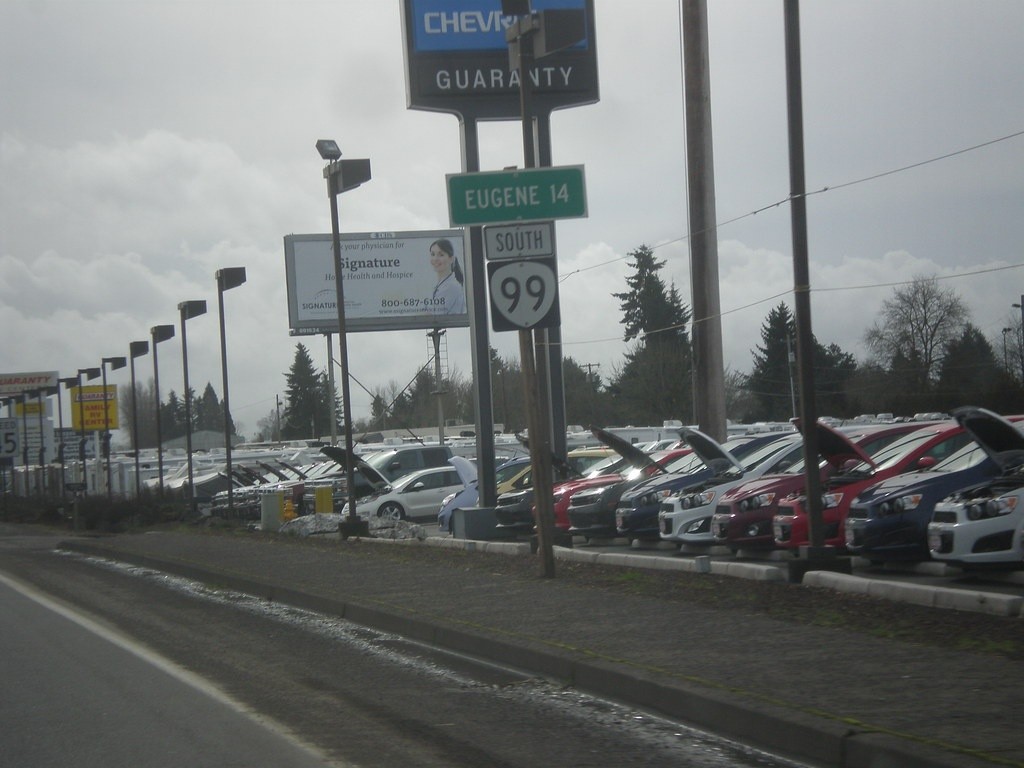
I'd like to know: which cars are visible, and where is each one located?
[0,407,1024,571]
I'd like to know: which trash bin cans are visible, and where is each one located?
[315,486,334,512]
[280,483,305,516]
[258,489,284,531]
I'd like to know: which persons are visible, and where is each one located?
[430,239,466,314]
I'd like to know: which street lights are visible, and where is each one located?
[79,368,103,493]
[0,377,79,517]
[130,341,149,500]
[101,357,127,500]
[150,324,176,497]
[177,299,207,503]
[316,138,371,529]
[215,266,247,516]
